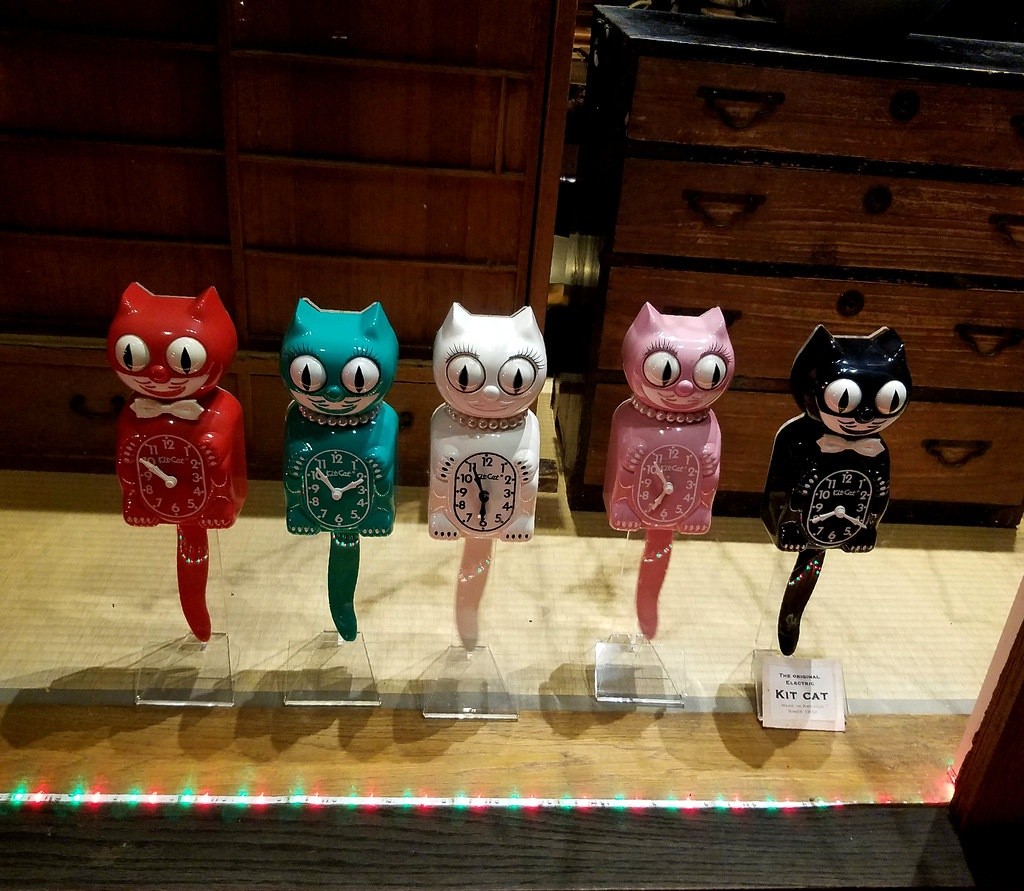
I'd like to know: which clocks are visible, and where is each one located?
[603,302,735,640]
[108,282,249,644]
[274,295,397,642]
[760,322,911,655]
[431,300,547,653]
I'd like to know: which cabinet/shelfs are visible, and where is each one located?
[548,0,1024,529]
[0,0,578,484]
